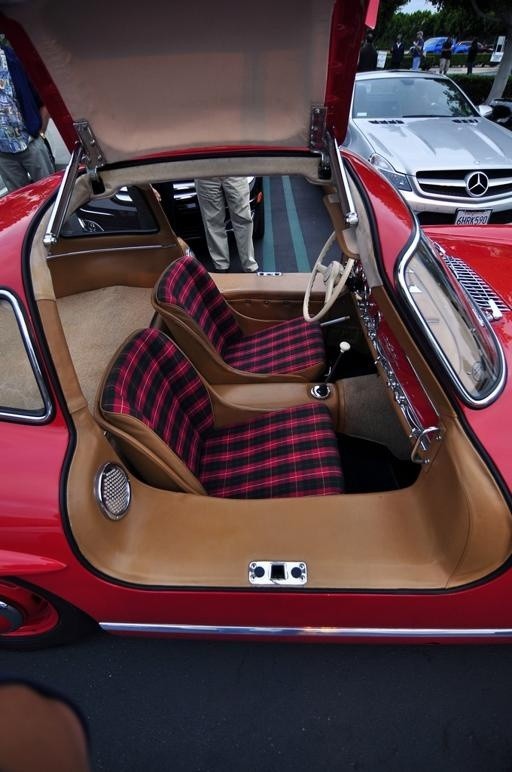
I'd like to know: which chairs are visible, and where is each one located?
[91,327,345,499]
[150,253,327,385]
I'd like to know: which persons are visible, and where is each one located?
[439,33,453,77]
[465,37,478,75]
[357,33,378,73]
[1,40,56,197]
[388,34,405,70]
[409,31,424,72]
[191,174,262,275]
